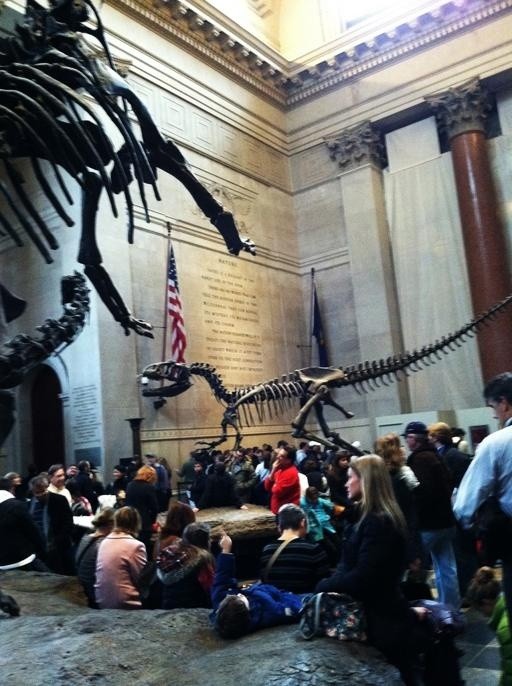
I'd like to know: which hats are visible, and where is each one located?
[144,450,159,459]
[398,423,430,437]
[181,524,210,549]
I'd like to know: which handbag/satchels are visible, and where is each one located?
[295,590,370,646]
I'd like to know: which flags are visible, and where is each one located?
[167,243,187,362]
[312,283,330,366]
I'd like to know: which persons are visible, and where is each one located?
[0,371,512,686]
[457,565,512,686]
